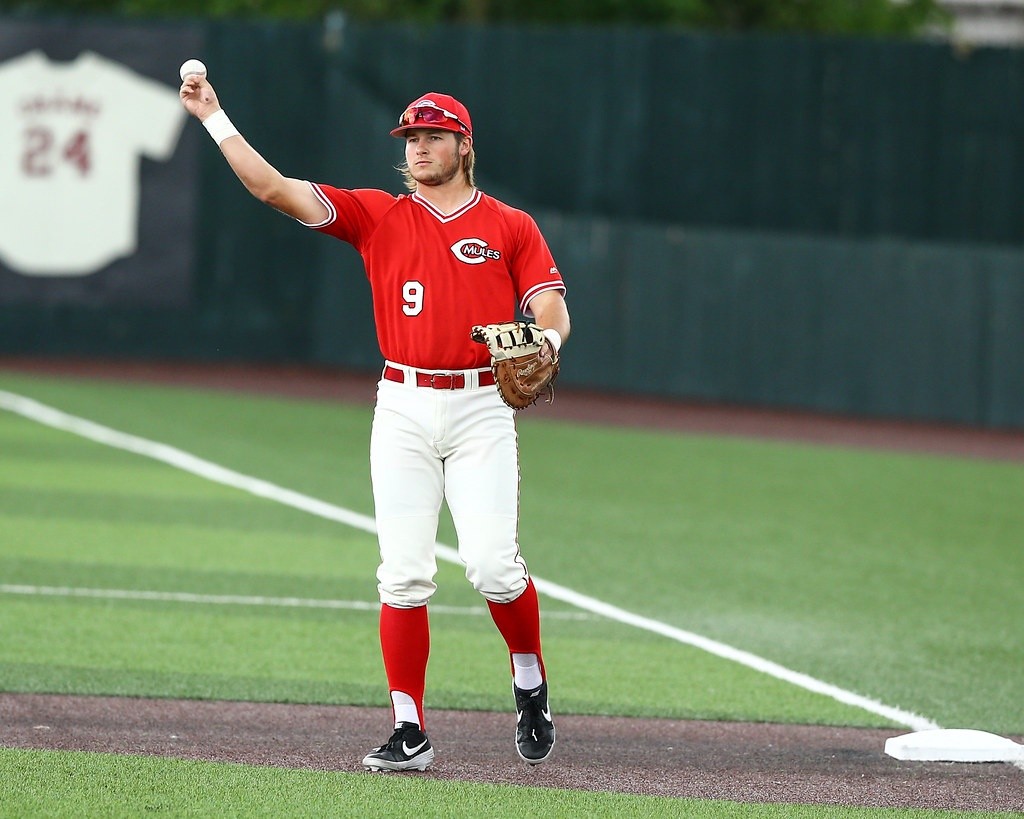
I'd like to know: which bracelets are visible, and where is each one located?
[542,329,562,353]
[202,109,240,147]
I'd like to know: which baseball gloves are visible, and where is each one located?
[468,319,561,410]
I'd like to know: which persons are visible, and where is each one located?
[180,73,572,772]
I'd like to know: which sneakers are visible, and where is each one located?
[512,679,555,763]
[361,721,434,773]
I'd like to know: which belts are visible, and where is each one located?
[384,365,496,389]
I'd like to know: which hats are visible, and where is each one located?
[389,92,472,138]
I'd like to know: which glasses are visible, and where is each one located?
[399,105,472,134]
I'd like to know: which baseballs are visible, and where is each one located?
[179,58,208,81]
